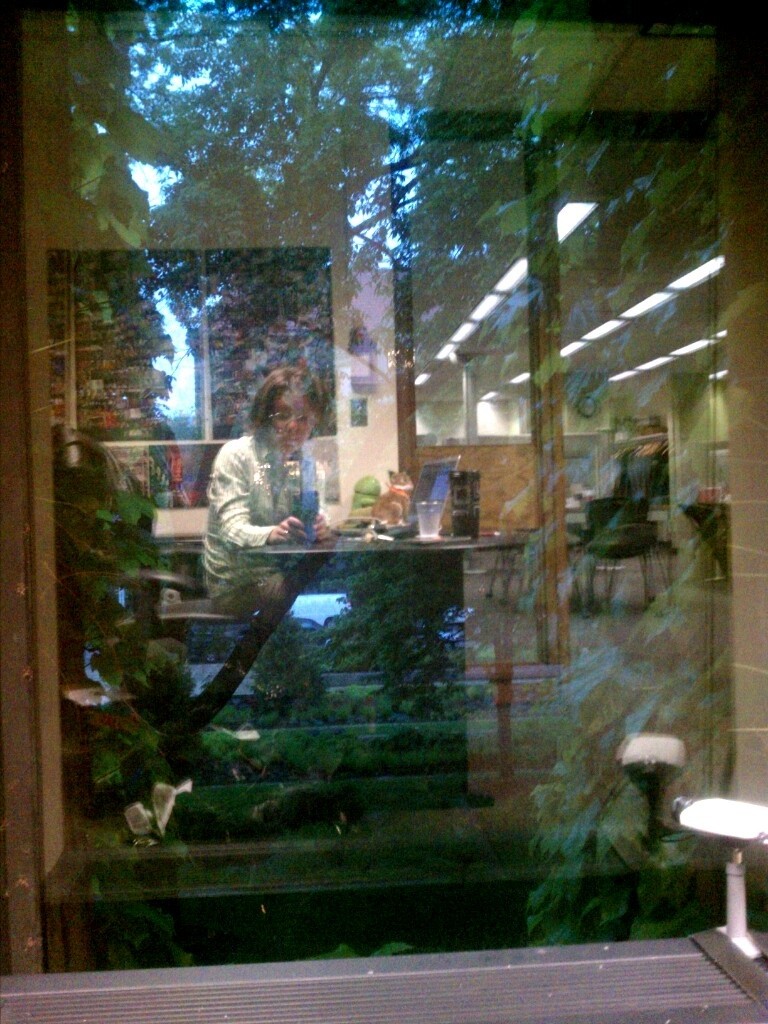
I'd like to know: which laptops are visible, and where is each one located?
[382,455,462,537]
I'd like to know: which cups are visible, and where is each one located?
[415,501,440,537]
[449,469,479,539]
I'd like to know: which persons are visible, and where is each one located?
[203,366,335,625]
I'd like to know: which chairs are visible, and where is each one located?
[69,442,361,827]
[491,499,729,616]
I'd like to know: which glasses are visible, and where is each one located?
[267,413,312,425]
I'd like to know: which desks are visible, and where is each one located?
[242,533,529,809]
